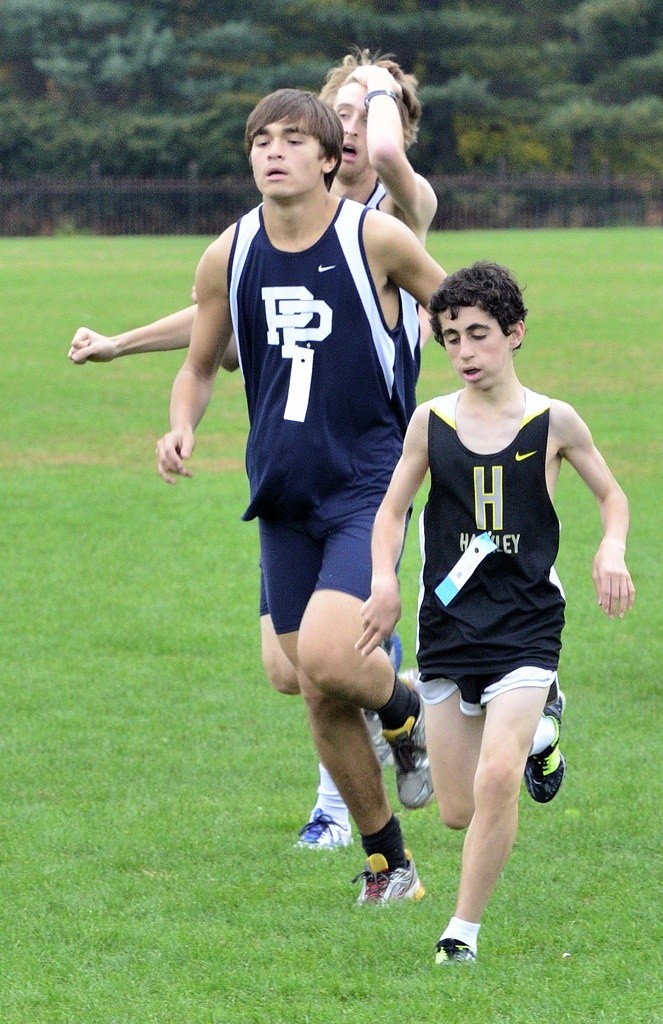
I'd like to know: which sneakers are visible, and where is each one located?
[381,670,436,809]
[524,689,565,803]
[351,849,426,909]
[434,938,477,966]
[359,632,402,763]
[292,807,354,851]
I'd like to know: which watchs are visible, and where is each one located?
[364,89,397,112]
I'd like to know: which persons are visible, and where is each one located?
[67,48,438,851]
[152,88,446,910]
[352,260,636,967]
